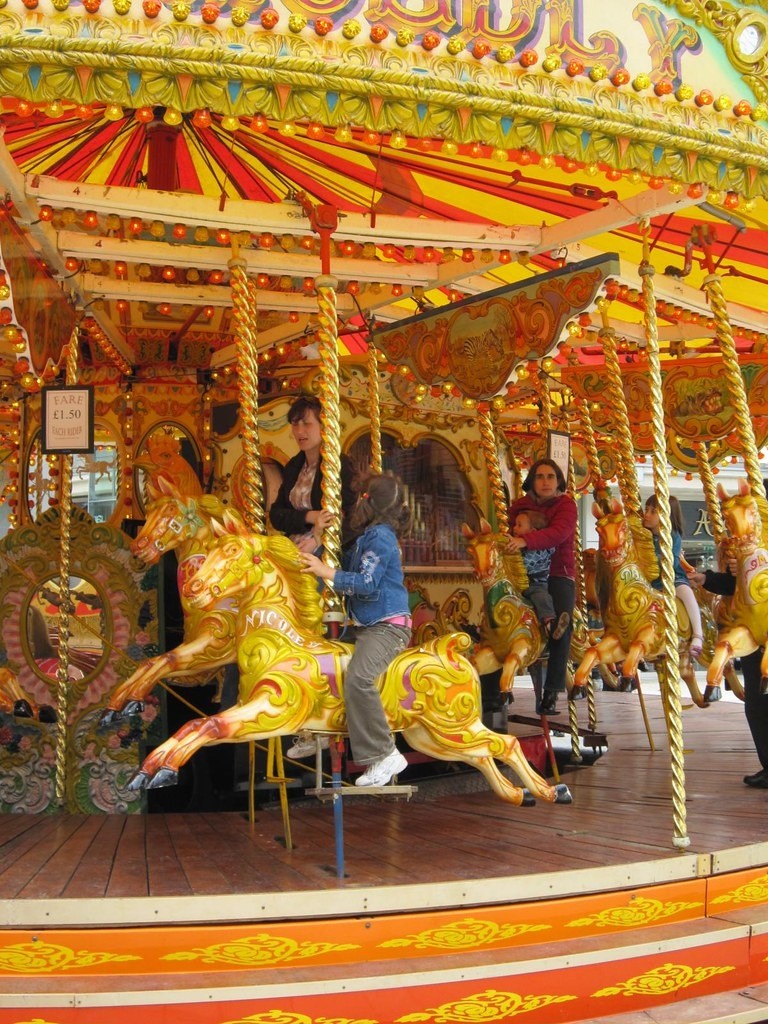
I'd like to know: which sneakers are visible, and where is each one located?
[286,737,331,759]
[355,747,408,788]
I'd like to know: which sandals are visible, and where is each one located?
[689,634,702,656]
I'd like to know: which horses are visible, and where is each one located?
[95,474,572,810]
[458,478,768,706]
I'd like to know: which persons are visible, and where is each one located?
[686,478,768,789]
[643,494,702,653]
[288,474,412,786]
[459,511,570,641]
[270,395,363,758]
[479,458,578,715]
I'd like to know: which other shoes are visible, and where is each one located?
[744,770,768,787]
[540,689,557,712]
[550,612,570,639]
